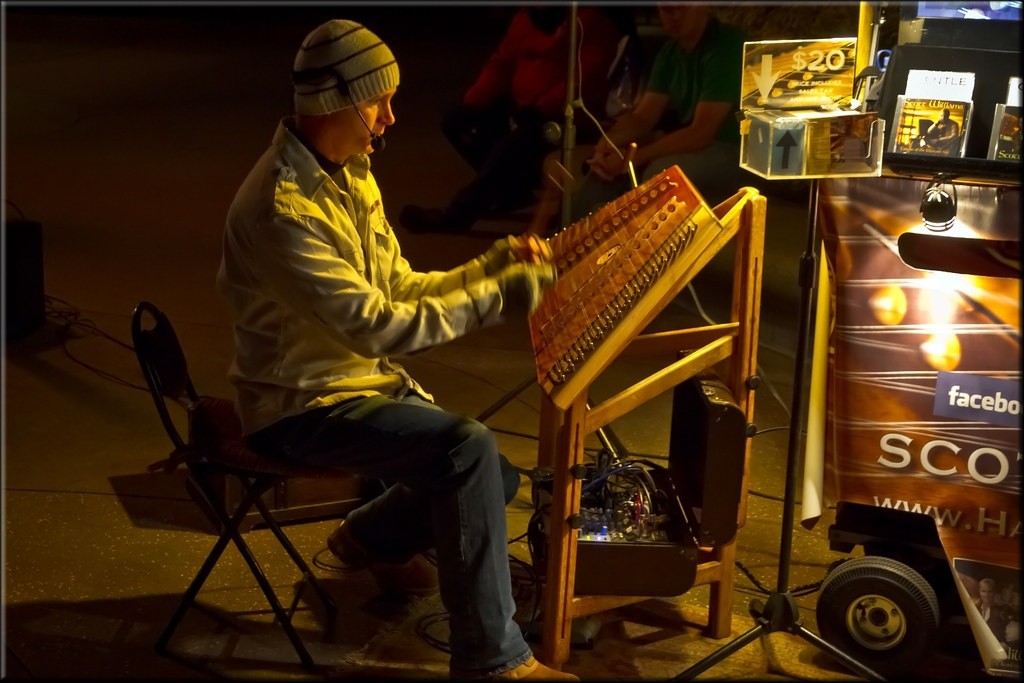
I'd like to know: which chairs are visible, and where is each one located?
[130,307,343,678]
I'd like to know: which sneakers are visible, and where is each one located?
[327,520,437,593]
[449,653,580,682]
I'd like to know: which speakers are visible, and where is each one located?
[4,218,47,340]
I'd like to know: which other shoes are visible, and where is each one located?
[399,204,466,233]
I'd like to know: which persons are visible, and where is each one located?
[215,18,580,679]
[927,108,959,149]
[440,1,620,216]
[577,2,752,218]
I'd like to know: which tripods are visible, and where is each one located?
[667,173,887,683]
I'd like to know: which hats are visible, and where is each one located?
[293,20,401,115]
